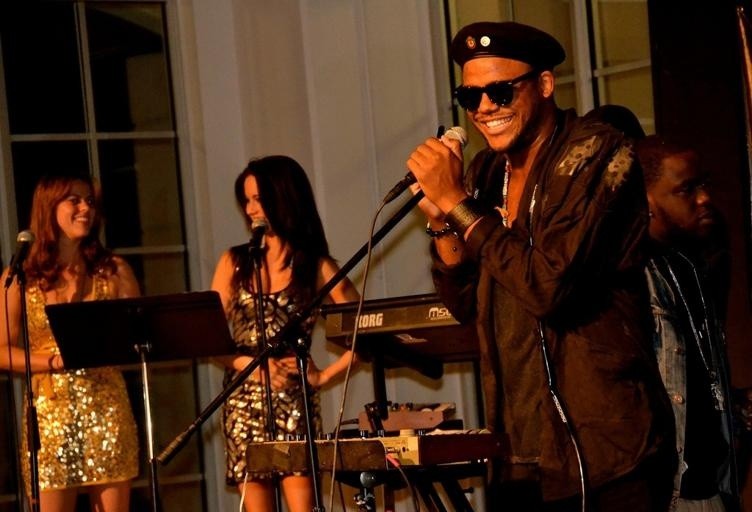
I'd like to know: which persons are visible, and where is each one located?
[406,19,680,511]
[1,168,143,511]
[632,131,745,511]
[210,154,365,512]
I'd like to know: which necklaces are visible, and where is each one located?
[494,154,511,228]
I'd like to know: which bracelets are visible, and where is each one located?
[423,220,457,237]
[443,195,486,235]
[311,368,320,389]
[48,355,58,371]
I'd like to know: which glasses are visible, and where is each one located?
[453,69,538,111]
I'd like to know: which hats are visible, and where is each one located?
[451,22,566,70]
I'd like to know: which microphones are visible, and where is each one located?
[235,220,269,271]
[5,230,36,288]
[381,125,470,206]
